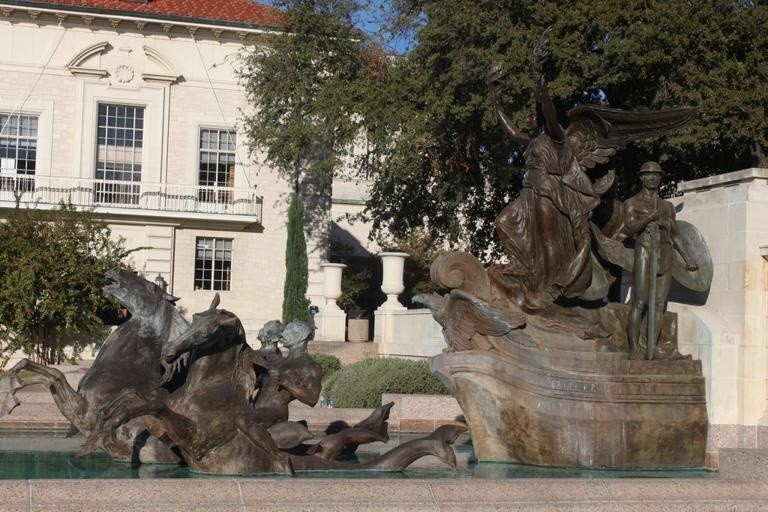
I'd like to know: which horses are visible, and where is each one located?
[75,292,276,477]
[0,266,193,464]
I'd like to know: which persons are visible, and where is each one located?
[491,63,599,311]
[243,316,283,407]
[620,157,698,361]
[236,318,323,476]
